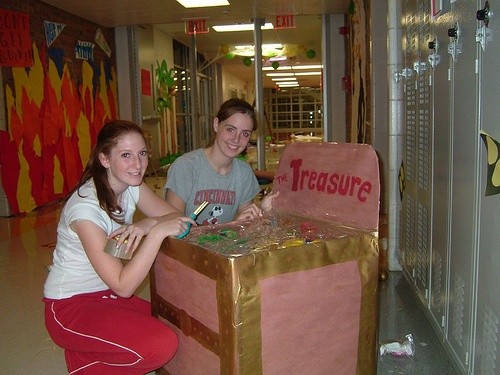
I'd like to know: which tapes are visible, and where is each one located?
[103,239,135,262]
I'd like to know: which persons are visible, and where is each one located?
[164,97,280,225]
[43,118,196,374]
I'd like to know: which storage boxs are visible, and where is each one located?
[153,214,378,374]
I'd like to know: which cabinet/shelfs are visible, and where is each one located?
[399,0,499,375]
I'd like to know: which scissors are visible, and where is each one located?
[175,201,209,240]
